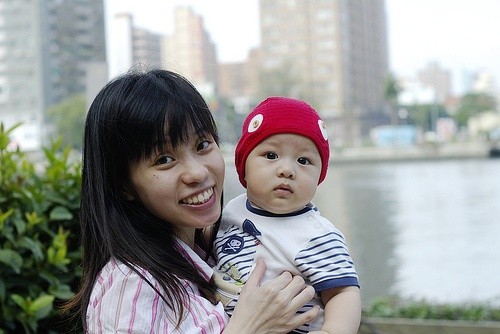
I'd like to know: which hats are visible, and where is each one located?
[235,97,330,183]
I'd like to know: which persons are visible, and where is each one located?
[197,96,364,334]
[60,71,321,334]
